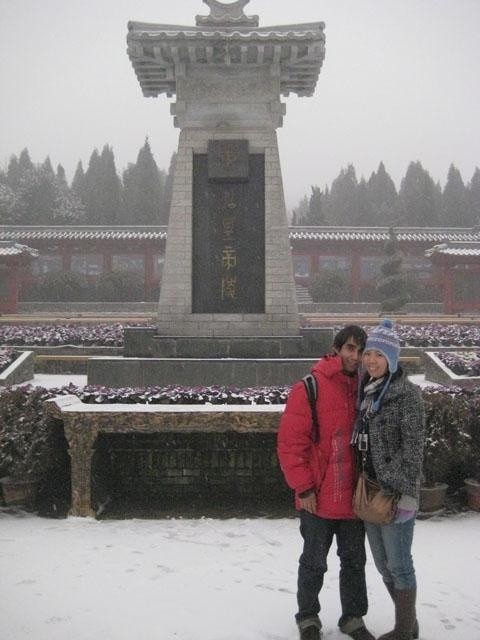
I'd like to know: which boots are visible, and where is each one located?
[378,582,419,640]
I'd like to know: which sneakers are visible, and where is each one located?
[348,626,375,640]
[300,625,320,640]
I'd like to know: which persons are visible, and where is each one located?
[351,319,427,640]
[277,326,373,640]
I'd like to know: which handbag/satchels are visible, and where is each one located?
[352,473,400,526]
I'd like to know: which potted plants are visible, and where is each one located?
[414,387,479,515]
[0,394,53,510]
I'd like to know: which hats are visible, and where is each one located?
[355,320,400,412]
